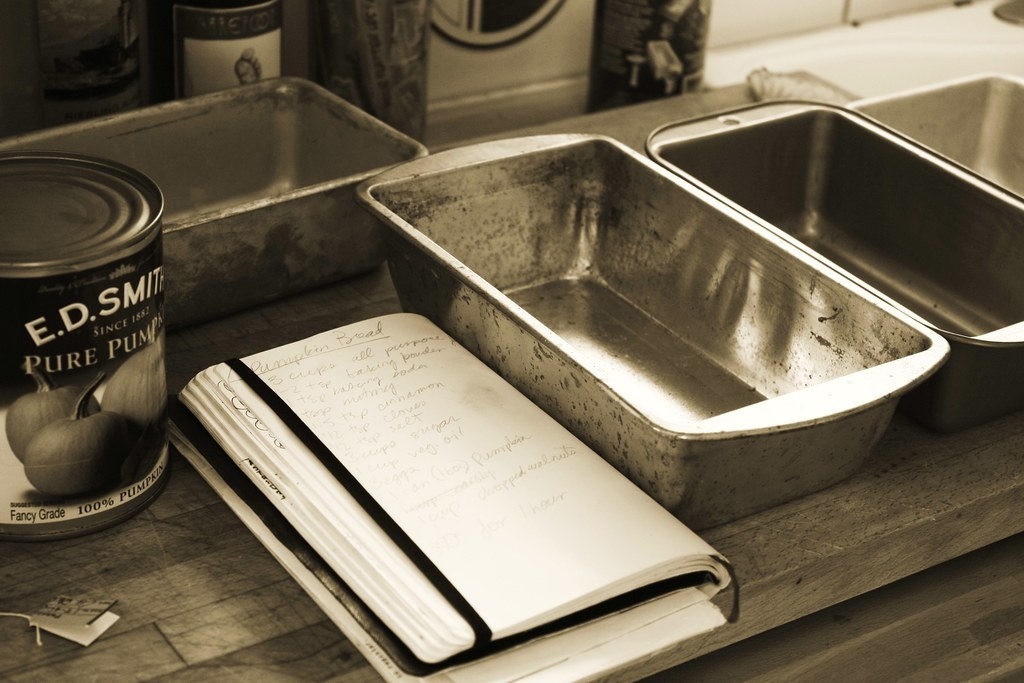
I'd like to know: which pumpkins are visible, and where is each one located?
[6,324,164,496]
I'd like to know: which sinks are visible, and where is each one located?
[852,76,1024,199]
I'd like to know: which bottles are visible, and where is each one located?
[585,0,711,111]
[149,0,282,105]
[307,1,433,143]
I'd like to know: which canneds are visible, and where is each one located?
[144,1,284,102]
[0,150,169,542]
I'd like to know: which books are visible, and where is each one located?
[166,312,740,683]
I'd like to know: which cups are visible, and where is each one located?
[34,0,140,126]
